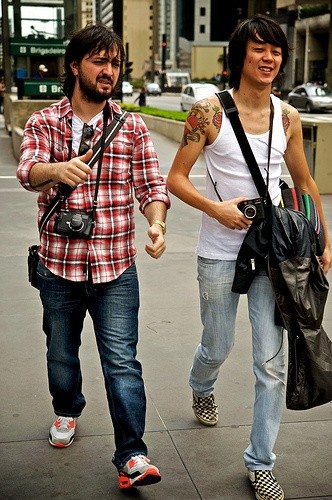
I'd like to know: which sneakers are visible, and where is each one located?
[48,416,80,448]
[118,453,162,491]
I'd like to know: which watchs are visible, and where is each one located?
[150,221,166,235]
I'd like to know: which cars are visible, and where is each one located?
[143,83,162,96]
[180,84,220,111]
[122,82,134,96]
[288,83,332,113]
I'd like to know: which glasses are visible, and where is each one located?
[76,122,95,161]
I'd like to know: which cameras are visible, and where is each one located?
[53,210,94,238]
[238,198,266,220]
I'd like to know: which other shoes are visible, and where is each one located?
[248,468,285,500]
[192,391,219,426]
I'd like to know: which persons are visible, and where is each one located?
[0,76,7,113]
[18,23,171,490]
[164,14,332,500]
[33,63,49,77]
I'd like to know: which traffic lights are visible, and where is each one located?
[126,62,133,74]
[222,69,228,78]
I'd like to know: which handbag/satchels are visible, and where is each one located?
[27,244,42,289]
[277,185,326,257]
